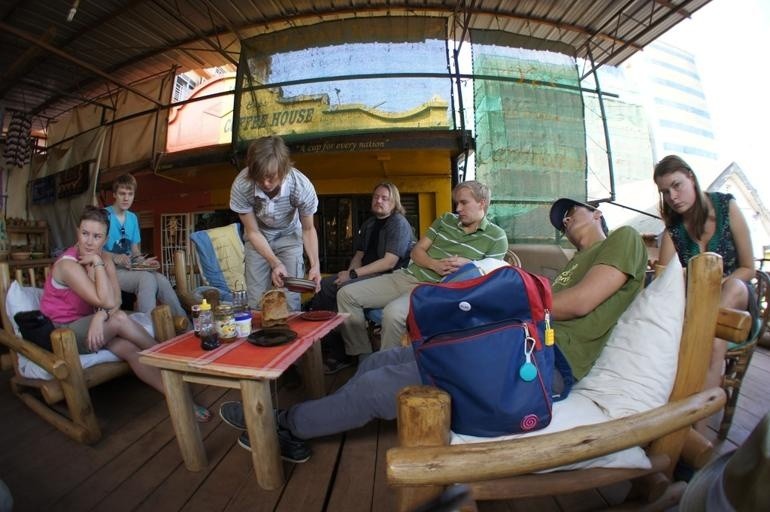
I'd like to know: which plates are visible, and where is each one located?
[300,309,338,321]
[244,328,298,347]
[130,268,156,272]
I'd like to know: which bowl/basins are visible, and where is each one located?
[29,253,48,259]
[11,252,32,260]
[279,275,316,295]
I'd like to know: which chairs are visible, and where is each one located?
[382,250,760,508]
[2,252,191,448]
[267,246,384,369]
[719,265,769,440]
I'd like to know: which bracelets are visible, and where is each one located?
[96,307,110,321]
[92,261,105,268]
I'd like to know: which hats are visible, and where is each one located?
[550,198,603,233]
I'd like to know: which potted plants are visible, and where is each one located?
[3,217,49,259]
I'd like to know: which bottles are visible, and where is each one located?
[230,290,251,316]
[190,303,203,337]
[197,299,220,351]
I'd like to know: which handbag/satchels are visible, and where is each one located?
[15,310,55,353]
[407,265,555,437]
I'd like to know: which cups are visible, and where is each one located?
[251,311,262,329]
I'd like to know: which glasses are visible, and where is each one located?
[85,203,110,220]
[121,227,126,247]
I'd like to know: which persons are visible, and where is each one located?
[101,173,193,333]
[39,204,212,423]
[412,408,770,512]
[229,132,322,311]
[335,181,509,370]
[218,196,648,465]
[313,179,417,372]
[646,152,759,433]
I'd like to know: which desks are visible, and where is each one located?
[136,306,352,491]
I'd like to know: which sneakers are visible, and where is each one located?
[323,357,352,374]
[219,401,312,463]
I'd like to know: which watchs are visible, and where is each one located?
[349,269,359,280]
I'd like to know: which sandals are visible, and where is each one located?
[191,405,211,423]
